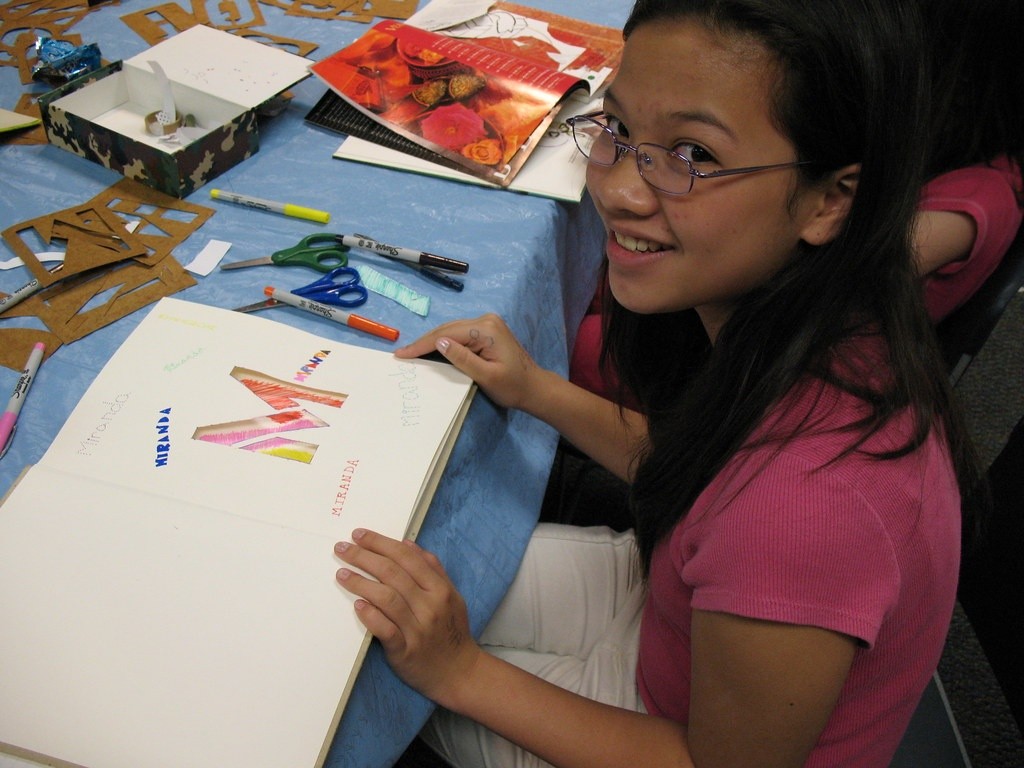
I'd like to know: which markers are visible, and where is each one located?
[341,235,469,272]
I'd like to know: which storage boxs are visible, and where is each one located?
[37,23,320,199]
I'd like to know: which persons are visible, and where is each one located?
[334,0,961,767]
[569,2,1023,418]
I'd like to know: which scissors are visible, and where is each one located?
[220,233,348,275]
[232,267,368,317]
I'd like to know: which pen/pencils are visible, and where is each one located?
[354,233,465,292]
[263,286,400,342]
[0,263,64,315]
[0,340,46,464]
[210,188,331,225]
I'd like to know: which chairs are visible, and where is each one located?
[936,227,1024,385]
[889,417,1024,767]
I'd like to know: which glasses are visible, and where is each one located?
[566,109,816,196]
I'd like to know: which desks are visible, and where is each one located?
[0,2,635,768]
